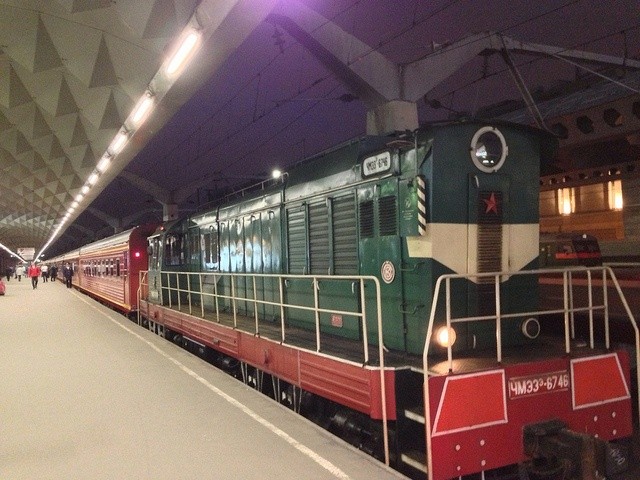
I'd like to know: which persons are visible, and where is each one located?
[6,266,11,281]
[64,262,73,288]
[50,266,56,281]
[28,262,40,289]
[0,278,5,295]
[41,263,48,283]
[16,266,23,281]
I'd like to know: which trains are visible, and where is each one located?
[36,115,640,480]
[540,232,603,274]
